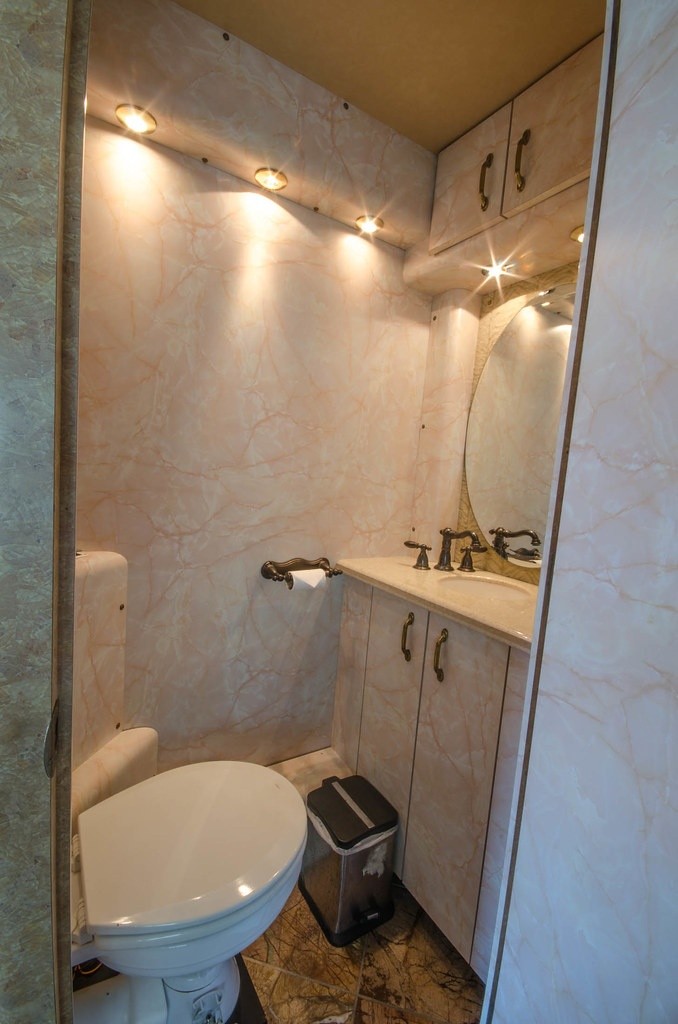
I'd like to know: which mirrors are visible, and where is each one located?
[465,282,576,569]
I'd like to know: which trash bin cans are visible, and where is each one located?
[297,773,402,948]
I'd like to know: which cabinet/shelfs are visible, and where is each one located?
[356,587,510,965]
[428,33,604,255]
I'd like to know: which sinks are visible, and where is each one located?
[436,574,531,601]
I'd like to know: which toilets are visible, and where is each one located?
[74,549,310,1023]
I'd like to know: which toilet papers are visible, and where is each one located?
[286,569,329,592]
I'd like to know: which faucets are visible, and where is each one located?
[434,526,480,572]
[489,526,541,561]
